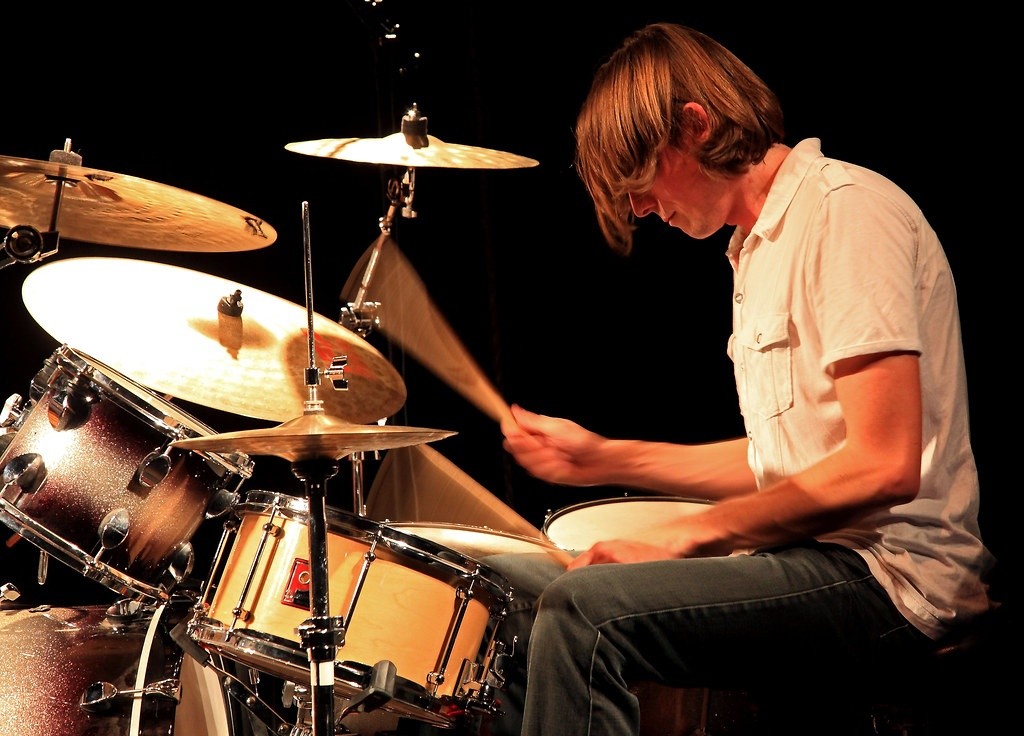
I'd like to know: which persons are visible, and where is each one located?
[472,22,994,736]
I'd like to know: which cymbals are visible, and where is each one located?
[0,158,282,258]
[167,410,460,462]
[283,131,540,173]
[22,255,411,423]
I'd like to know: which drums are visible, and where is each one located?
[0,596,236,736]
[0,341,255,604]
[543,493,766,736]
[185,489,514,729]
[383,520,570,566]
